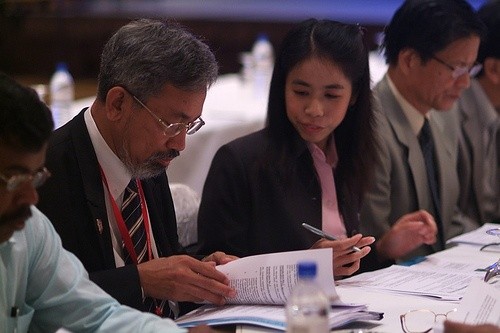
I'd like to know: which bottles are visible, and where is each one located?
[251,32,275,95]
[50,61,74,131]
[284,262,330,333]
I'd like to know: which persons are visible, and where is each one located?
[31,16,244,320]
[365,0,482,264]
[196,18,437,282]
[460,0,500,225]
[0,76,188,333]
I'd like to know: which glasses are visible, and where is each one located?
[0,167,50,191]
[428,53,482,80]
[130,94,205,138]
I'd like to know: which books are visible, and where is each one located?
[175,247,384,331]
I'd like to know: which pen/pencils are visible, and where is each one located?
[11,307,19,333]
[302,223,361,252]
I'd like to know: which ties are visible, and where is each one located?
[419,116,446,250]
[120,176,176,318]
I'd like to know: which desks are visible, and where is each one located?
[50,50,389,195]
